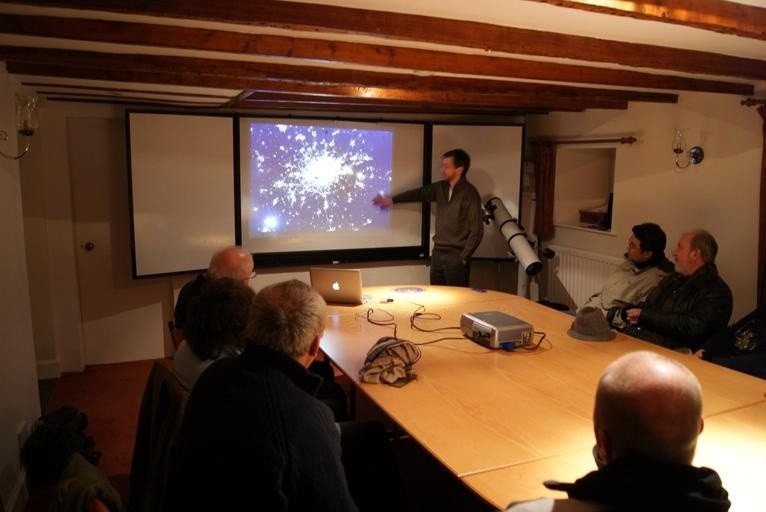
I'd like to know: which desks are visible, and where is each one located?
[323,284,765,512]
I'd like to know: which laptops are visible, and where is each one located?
[310,267,369,304]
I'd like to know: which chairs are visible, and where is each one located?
[126,359,192,491]
[169,446,293,512]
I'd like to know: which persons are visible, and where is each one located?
[624,228,732,351]
[561,222,674,343]
[504,349,731,512]
[164,243,363,511]
[372,149,484,289]
[690,304,765,379]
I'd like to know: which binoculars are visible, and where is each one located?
[481,194,543,276]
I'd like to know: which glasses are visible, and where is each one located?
[628,238,640,251]
[245,269,257,281]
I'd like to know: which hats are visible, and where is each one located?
[567,306,617,341]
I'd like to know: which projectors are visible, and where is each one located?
[460,311,535,350]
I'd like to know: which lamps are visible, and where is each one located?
[0,78,38,163]
[667,123,702,173]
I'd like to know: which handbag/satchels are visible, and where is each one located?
[21,404,103,496]
